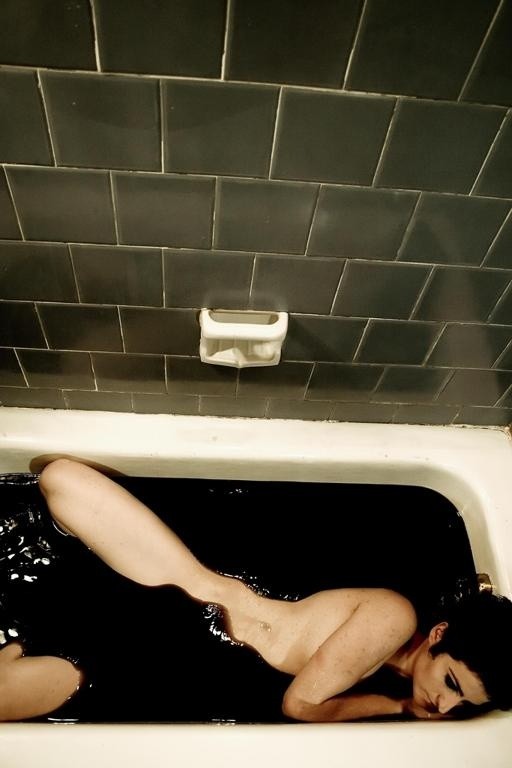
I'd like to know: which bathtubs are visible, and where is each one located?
[0,404,512,768]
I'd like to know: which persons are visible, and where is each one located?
[0,458,510,724]
